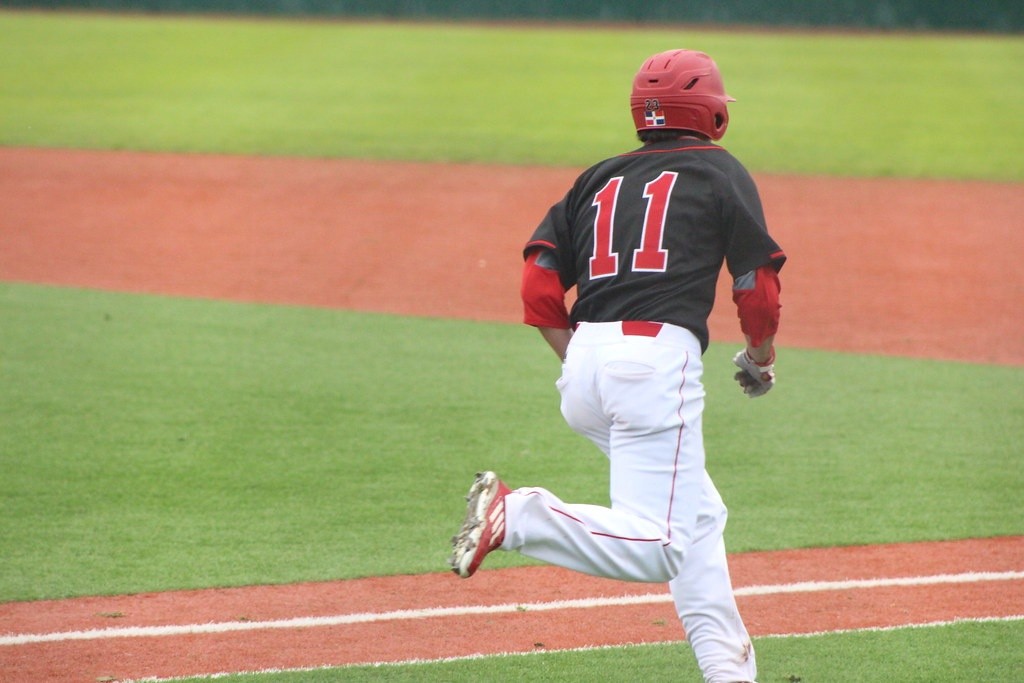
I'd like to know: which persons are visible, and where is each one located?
[449,45,788,679]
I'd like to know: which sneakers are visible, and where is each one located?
[450,470,513,576]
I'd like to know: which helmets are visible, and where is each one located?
[629,50,736,141]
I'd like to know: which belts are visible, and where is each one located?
[573,321,701,355]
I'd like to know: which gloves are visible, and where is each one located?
[732,344,775,398]
